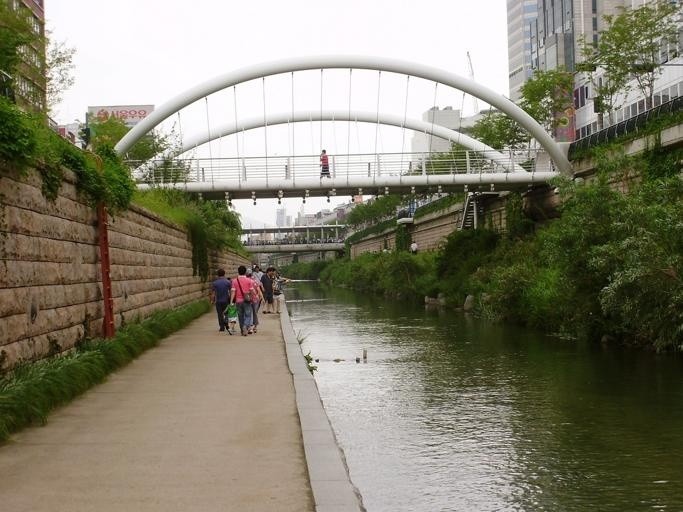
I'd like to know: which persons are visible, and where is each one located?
[317,149,329,176]
[208,265,289,337]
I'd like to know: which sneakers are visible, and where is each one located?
[263,311,282,314]
[241,328,257,336]
[219,326,236,335]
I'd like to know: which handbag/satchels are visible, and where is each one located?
[243,293,251,303]
